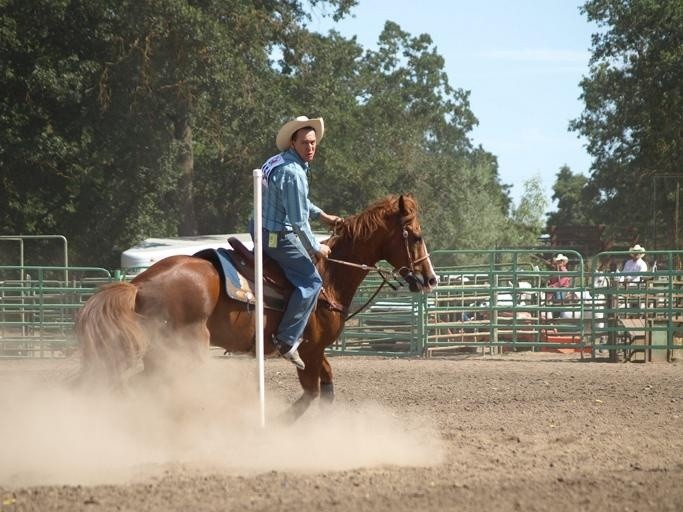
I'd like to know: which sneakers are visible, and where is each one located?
[273,337,305,369]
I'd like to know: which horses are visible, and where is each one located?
[73,191,438,426]
[479,269,668,354]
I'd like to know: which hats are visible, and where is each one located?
[276,115,325,151]
[628,245,646,258]
[553,254,568,265]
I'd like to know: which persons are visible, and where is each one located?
[247,115,346,371]
[457,244,650,355]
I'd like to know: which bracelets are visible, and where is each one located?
[333,217,340,226]
